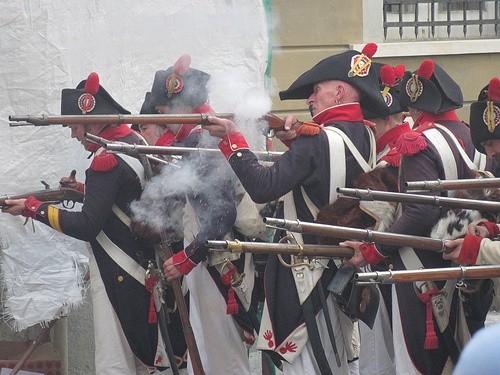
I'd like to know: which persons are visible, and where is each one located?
[1,72,171,375]
[130,43,500,375]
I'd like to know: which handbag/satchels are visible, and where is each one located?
[327,264,380,331]
[456,276,497,323]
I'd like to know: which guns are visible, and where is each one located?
[102,143,286,162]
[404,178,500,194]
[8,112,320,139]
[137,139,206,375]
[0,181,84,209]
[205,239,354,258]
[335,187,500,214]
[263,217,500,255]
[83,132,181,170]
[354,265,500,287]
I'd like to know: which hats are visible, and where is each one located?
[374,64,412,116]
[151,54,211,107]
[131,92,159,132]
[61,72,131,127]
[405,60,463,114]
[470,77,500,155]
[279,43,389,121]
